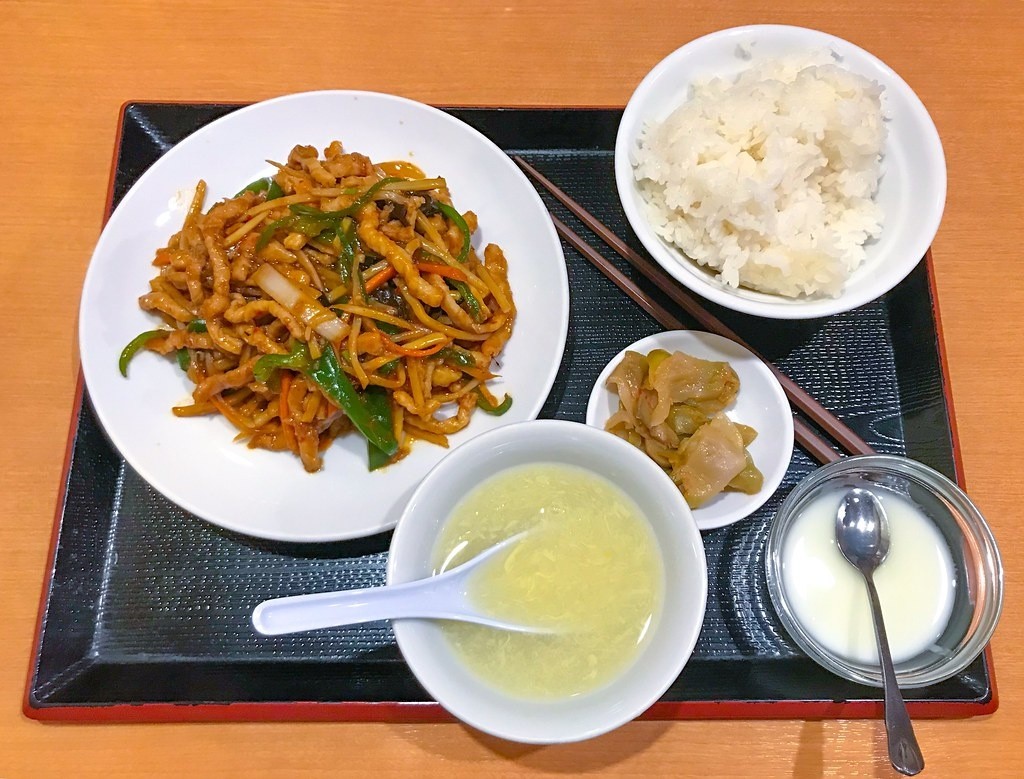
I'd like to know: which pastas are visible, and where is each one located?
[118,138,516,475]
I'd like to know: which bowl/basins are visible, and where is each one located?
[614,23,950,318]
[765,455,1004,689]
[386,420,708,744]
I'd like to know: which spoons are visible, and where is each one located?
[837,487,927,775]
[251,524,557,638]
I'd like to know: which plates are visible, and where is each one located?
[585,328,795,531]
[77,91,572,541]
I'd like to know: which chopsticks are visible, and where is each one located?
[514,155,881,467]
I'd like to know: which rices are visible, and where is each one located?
[632,40,894,302]
